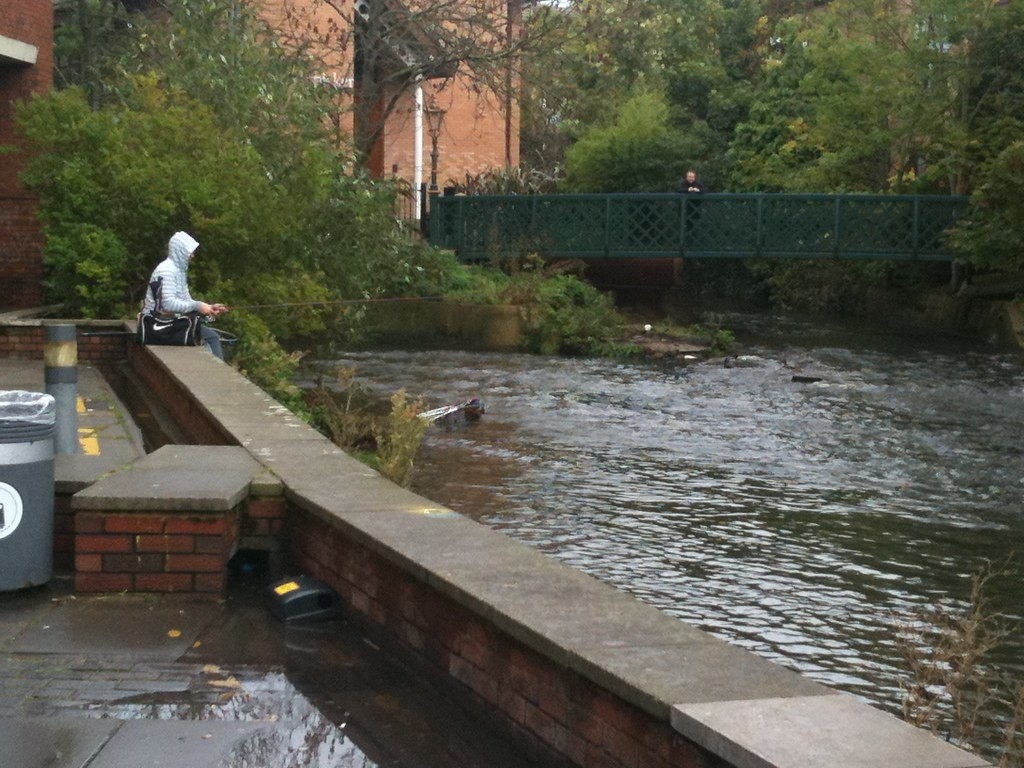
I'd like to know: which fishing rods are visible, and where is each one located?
[211,293,446,309]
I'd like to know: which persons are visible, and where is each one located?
[142,231,229,362]
[676,170,705,244]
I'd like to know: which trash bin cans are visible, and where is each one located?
[213,329,238,367]
[0,390,57,592]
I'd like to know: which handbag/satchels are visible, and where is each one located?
[139,276,201,346]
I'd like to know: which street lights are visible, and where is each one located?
[423,101,446,217]
